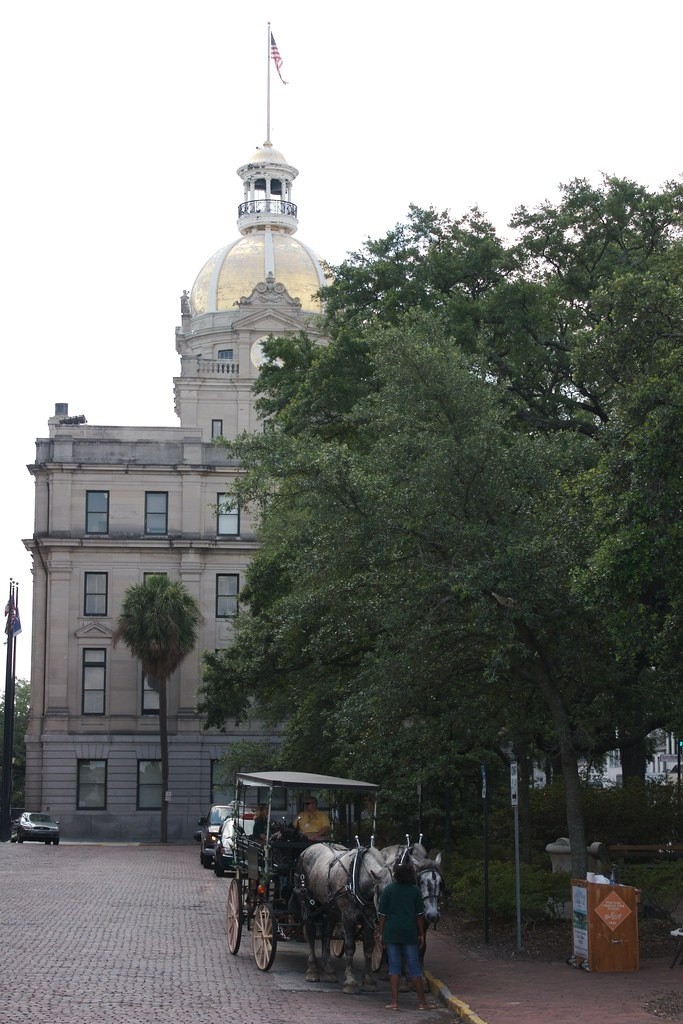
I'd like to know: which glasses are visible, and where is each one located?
[305,802,315,805]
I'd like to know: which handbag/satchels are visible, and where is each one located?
[269,831,282,841]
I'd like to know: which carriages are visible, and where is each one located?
[227,770,444,995]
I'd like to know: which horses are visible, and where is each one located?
[289,842,442,994]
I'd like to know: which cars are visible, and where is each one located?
[11,812,60,846]
[198,800,257,877]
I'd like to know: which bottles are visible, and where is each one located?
[610,870,615,885]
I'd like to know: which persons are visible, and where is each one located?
[180,289,191,315]
[293,796,331,840]
[253,803,268,841]
[378,862,439,1010]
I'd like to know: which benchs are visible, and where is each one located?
[667,895,683,969]
[599,838,683,864]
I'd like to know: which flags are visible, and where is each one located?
[3,594,22,645]
[271,32,288,85]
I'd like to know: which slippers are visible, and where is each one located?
[419,1004,437,1010]
[385,1004,399,1010]
